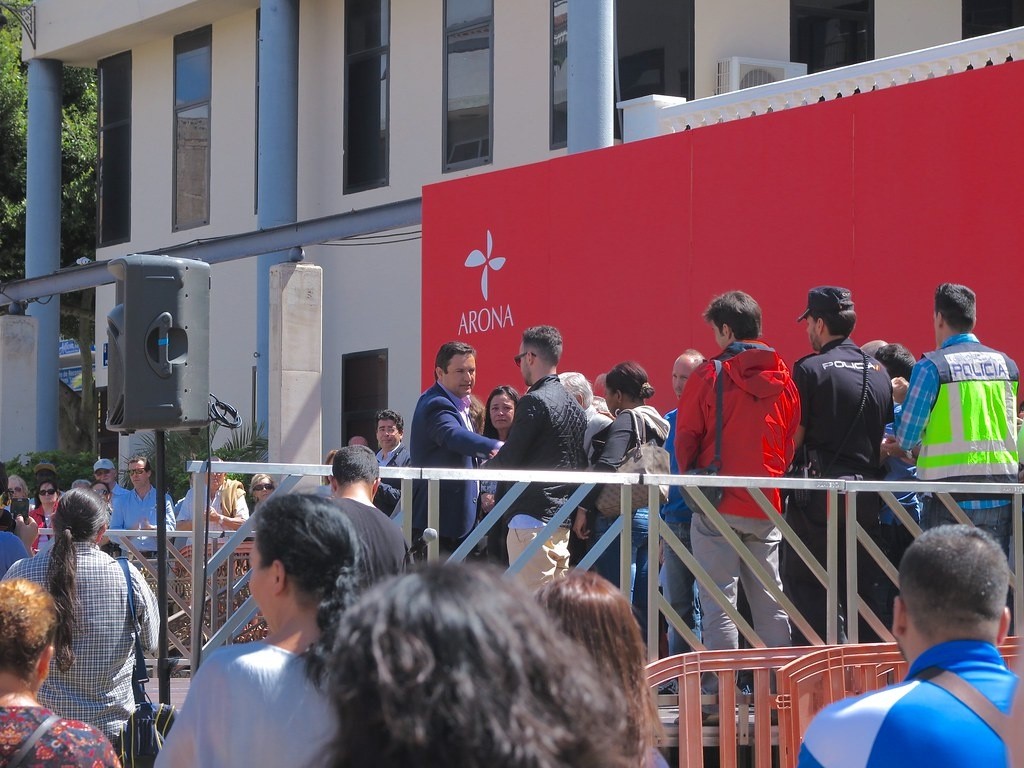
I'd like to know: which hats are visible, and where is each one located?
[93,459,115,475]
[796,285,853,323]
[34,461,57,474]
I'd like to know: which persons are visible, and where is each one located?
[0,287,1024,768]
[0,576,122,768]
[410,339,505,566]
[673,290,801,726]
[0,487,162,768]
[303,559,649,768]
[793,524,1024,768]
[890,283,1020,640]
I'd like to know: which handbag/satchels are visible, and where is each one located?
[116,556,178,768]
[595,408,671,517]
[678,359,722,514]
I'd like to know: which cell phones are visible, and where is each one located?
[795,444,809,508]
[10,499,29,527]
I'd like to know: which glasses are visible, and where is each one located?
[125,468,145,476]
[252,484,274,491]
[514,351,537,368]
[94,469,112,476]
[93,489,109,495]
[8,487,22,492]
[38,489,56,495]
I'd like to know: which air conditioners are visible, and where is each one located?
[715,57,807,94]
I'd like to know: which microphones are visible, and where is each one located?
[404,527,437,559]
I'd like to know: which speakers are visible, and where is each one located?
[106,253,211,436]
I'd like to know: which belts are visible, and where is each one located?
[139,551,157,558]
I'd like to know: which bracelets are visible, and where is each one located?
[217,515,224,524]
